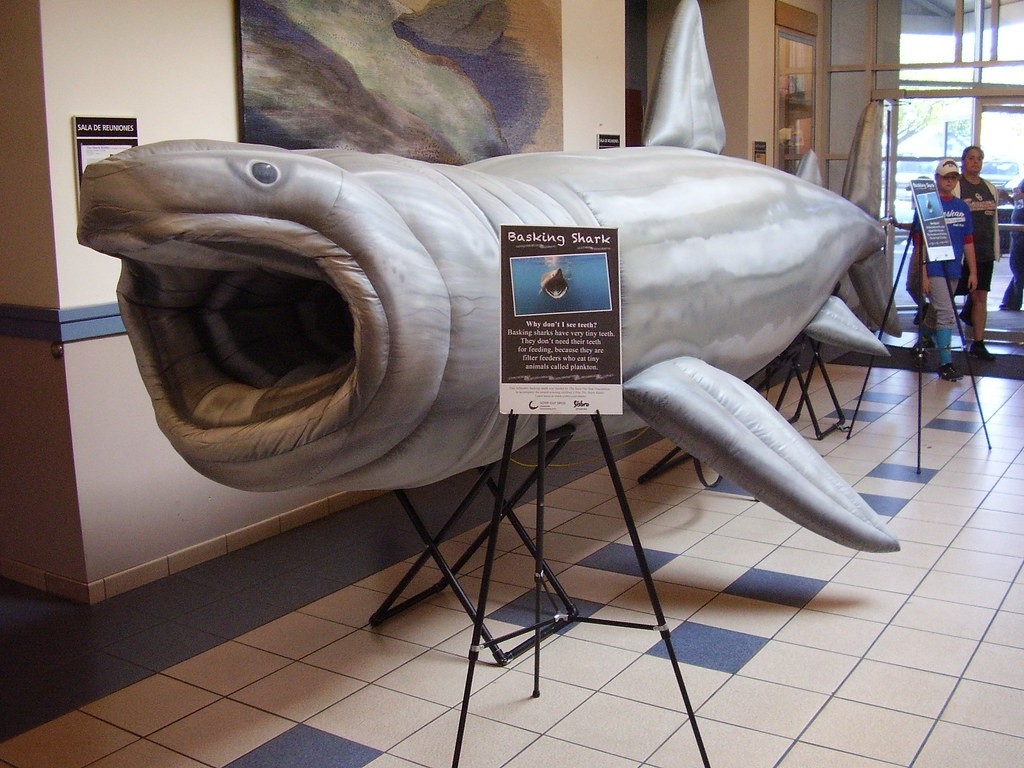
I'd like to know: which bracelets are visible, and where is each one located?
[922,260,927,264]
[899,223,902,229]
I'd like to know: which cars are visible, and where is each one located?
[980,159,1024,195]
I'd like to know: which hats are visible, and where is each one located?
[936,158,959,176]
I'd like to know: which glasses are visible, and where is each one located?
[941,175,957,180]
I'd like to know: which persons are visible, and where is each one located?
[998,178,1024,312]
[914,158,978,381]
[888,175,931,326]
[951,145,1002,361]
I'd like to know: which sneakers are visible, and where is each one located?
[969,340,995,361]
[913,341,935,348]
[937,365,963,381]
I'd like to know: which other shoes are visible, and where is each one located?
[999,304,1020,311]
[913,303,929,325]
